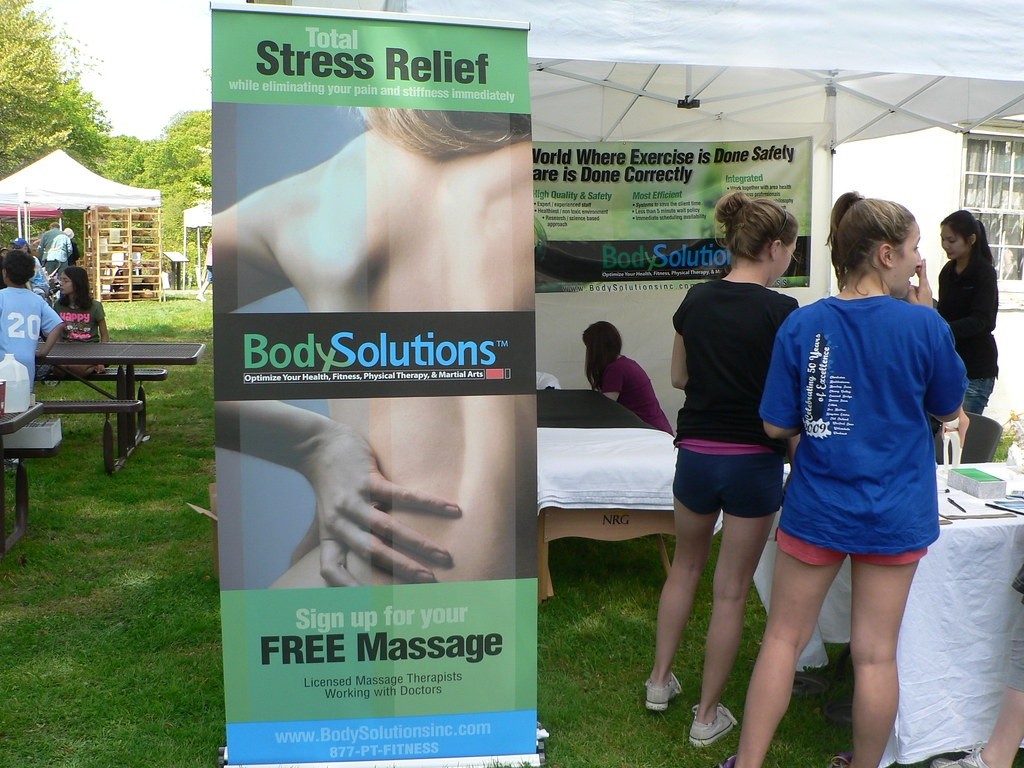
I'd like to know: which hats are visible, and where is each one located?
[10,237,27,247]
[63,227,74,239]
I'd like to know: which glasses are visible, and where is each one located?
[59,279,71,285]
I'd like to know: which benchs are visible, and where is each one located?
[33,368,168,415]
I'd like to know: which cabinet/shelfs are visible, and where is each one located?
[83,207,164,300]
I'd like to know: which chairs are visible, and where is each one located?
[933,412,1001,463]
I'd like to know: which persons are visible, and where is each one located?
[196,238,214,303]
[933,210,999,414]
[930,562,1024,768]
[645,192,802,748]
[0,223,110,473]
[214,106,536,586]
[581,321,674,438]
[718,190,970,768]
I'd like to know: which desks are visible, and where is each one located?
[0,401,46,555]
[24,341,204,472]
[538,387,725,602]
[752,468,1024,768]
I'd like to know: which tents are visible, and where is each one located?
[386,0,1024,439]
[0,149,163,302]
[183,203,213,291]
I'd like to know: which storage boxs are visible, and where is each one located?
[947,468,1006,500]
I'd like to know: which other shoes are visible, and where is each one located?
[718,756,737,768]
[196,292,206,302]
[831,751,854,767]
[930,740,991,768]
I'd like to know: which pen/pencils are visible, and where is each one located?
[985,503,1024,516]
[946,497,966,514]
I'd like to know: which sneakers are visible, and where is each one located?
[688,702,739,749]
[645,668,681,711]
